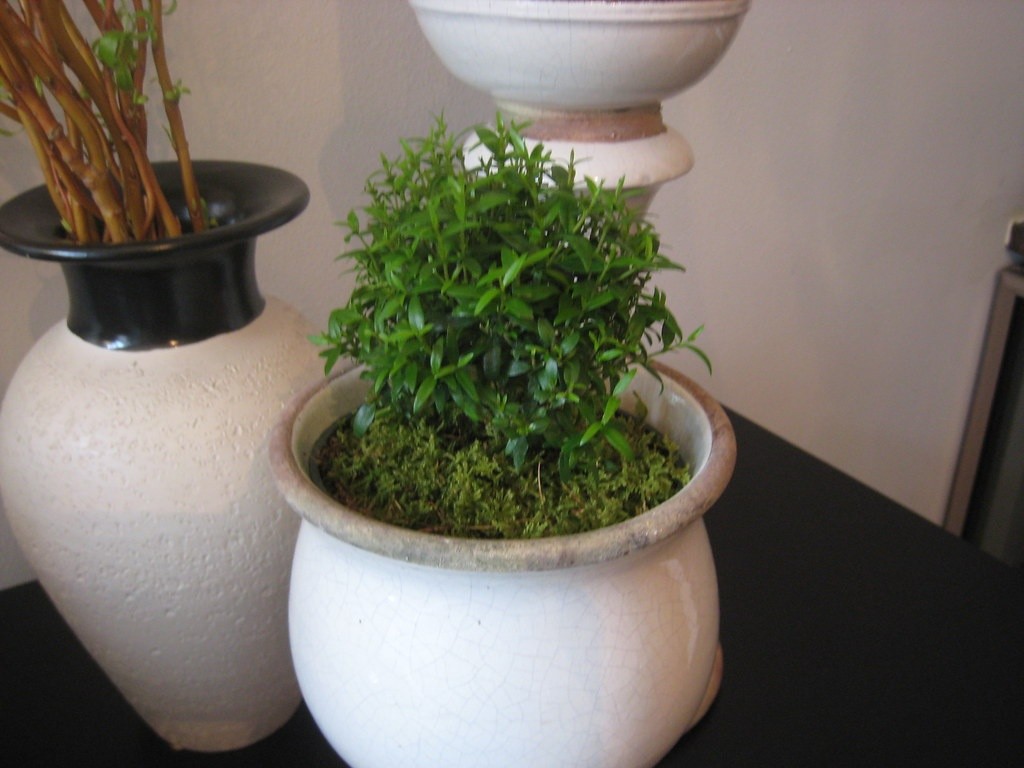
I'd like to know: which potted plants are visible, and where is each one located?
[0,0,330,752]
[270,107,736,767]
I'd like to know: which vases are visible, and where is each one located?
[408,0,752,190]
